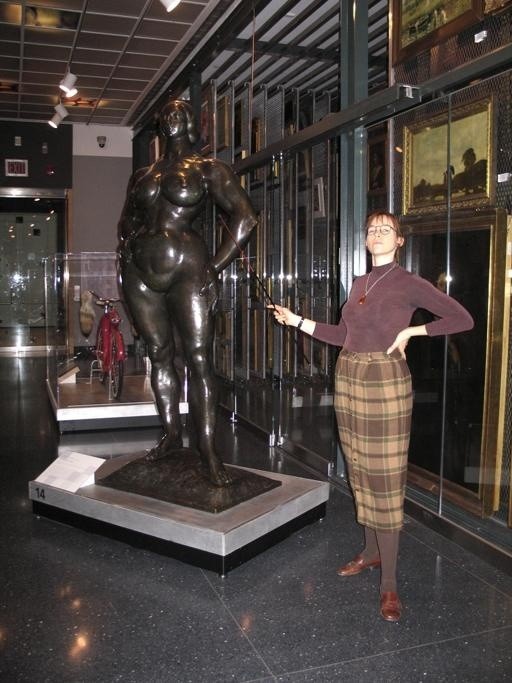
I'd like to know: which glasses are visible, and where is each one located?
[364,225,394,236]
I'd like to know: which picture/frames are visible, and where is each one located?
[388,68,511,214]
[393,208,511,522]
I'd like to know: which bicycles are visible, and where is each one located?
[84,290,127,402]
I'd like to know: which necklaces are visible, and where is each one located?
[356,261,397,305]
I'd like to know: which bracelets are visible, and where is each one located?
[297,317,305,330]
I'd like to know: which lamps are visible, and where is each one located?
[50,64,76,134]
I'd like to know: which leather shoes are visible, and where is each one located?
[379,585,402,621]
[335,552,380,576]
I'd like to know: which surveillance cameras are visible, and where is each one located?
[98,136,106,148]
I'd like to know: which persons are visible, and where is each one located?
[117,100,259,488]
[460,147,480,196]
[414,178,434,201]
[443,165,455,201]
[266,210,475,622]
